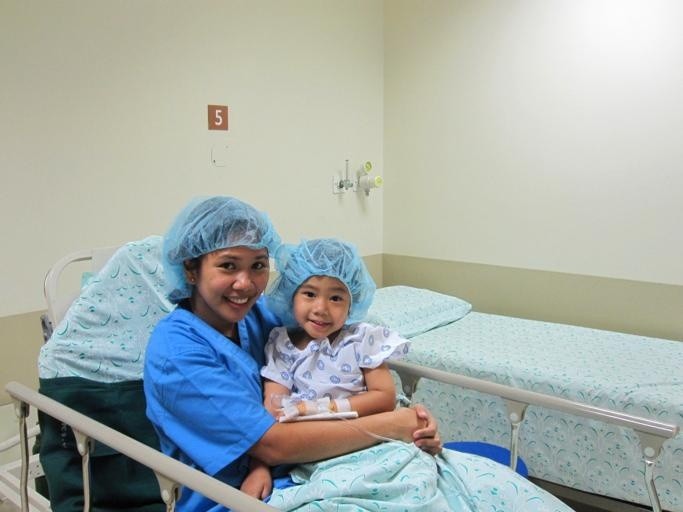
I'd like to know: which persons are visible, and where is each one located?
[144,195,445,512]
[240,238,413,503]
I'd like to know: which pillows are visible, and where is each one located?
[35,236,193,386]
[365,280,473,344]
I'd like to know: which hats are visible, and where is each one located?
[161,197,281,304]
[268,237,377,330]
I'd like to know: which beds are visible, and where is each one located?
[366,279,682,511]
[33,245,680,511]
